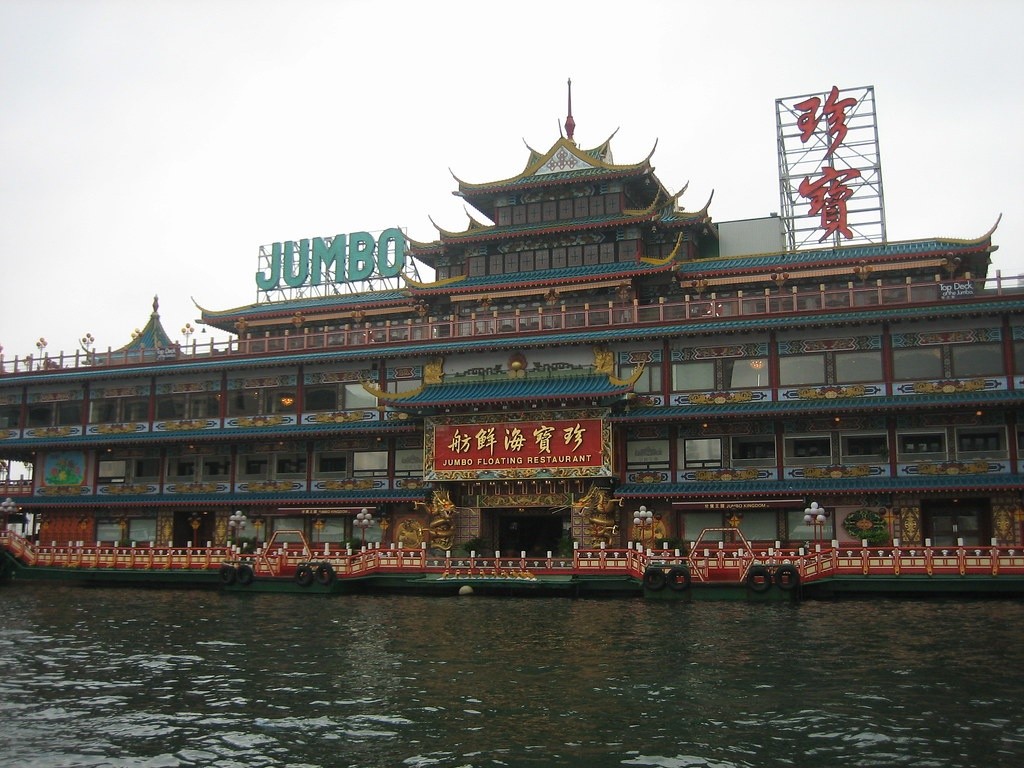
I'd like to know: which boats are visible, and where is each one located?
[1,78,1024,598]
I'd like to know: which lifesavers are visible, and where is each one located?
[236,565,253,585]
[295,566,314,587]
[747,566,772,593]
[775,564,800,591]
[643,568,666,592]
[219,566,236,586]
[316,564,333,585]
[668,567,691,591]
